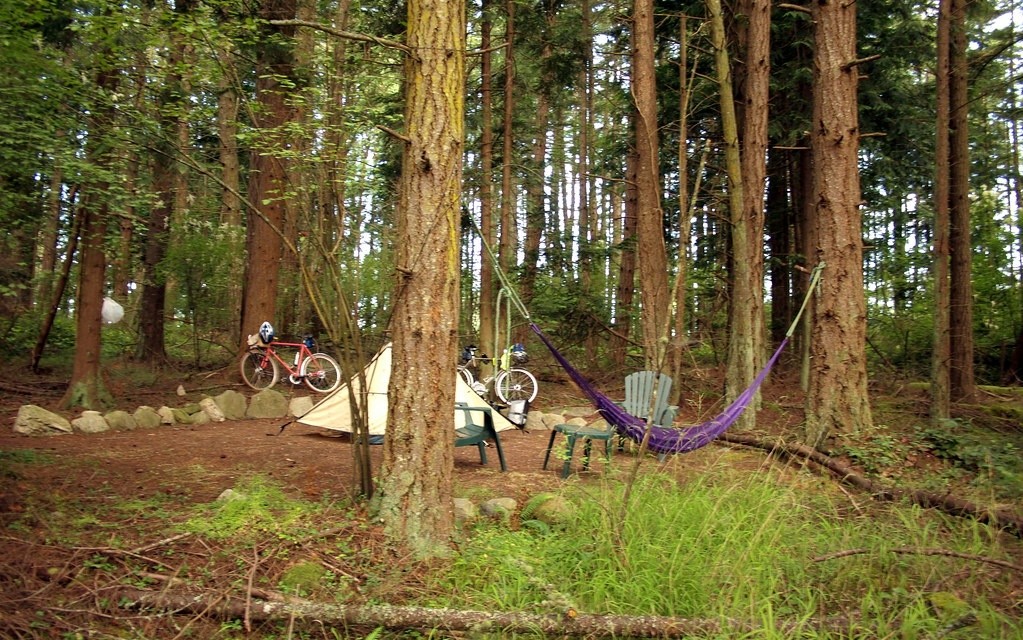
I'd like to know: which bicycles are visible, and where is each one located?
[457,345,539,405]
[239,322,341,393]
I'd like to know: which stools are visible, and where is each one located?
[543,424,611,481]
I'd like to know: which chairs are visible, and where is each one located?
[454,401,507,472]
[615,370,679,462]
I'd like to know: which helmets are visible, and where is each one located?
[259,321,273,344]
[510,351,529,364]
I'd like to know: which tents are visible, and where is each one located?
[296,339,528,444]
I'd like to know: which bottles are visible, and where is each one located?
[293,352,299,366]
